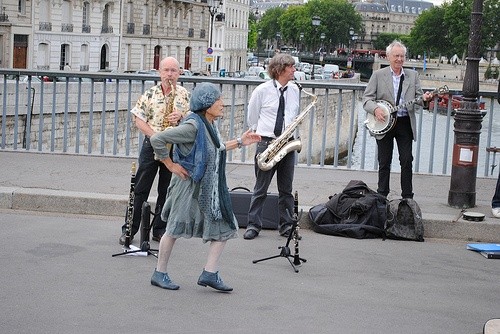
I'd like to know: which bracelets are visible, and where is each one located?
[237,137,242,149]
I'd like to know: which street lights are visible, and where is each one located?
[352,33,359,71]
[208,3,218,73]
[310,15,321,80]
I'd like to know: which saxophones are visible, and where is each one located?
[256,82,318,171]
[154,79,177,160]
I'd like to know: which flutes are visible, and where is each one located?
[293,191,301,266]
[120,162,136,249]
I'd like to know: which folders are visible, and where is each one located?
[466,244,500,259]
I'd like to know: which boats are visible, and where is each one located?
[428,94,488,117]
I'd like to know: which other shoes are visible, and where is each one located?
[492,207,500,218]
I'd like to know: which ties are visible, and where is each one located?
[273,85,289,138]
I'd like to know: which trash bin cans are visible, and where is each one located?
[220,69,225,77]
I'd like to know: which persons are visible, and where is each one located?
[294,69,300,81]
[362,41,432,200]
[150,82,261,292]
[64,63,71,72]
[119,56,193,245]
[491,79,500,218]
[243,53,303,240]
[299,69,305,80]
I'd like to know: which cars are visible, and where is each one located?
[4,54,340,83]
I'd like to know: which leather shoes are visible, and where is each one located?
[197,268,233,292]
[152,236,161,242]
[151,267,180,290]
[243,229,258,239]
[284,229,302,240]
[119,234,134,245]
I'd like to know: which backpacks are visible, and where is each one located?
[383,197,425,242]
